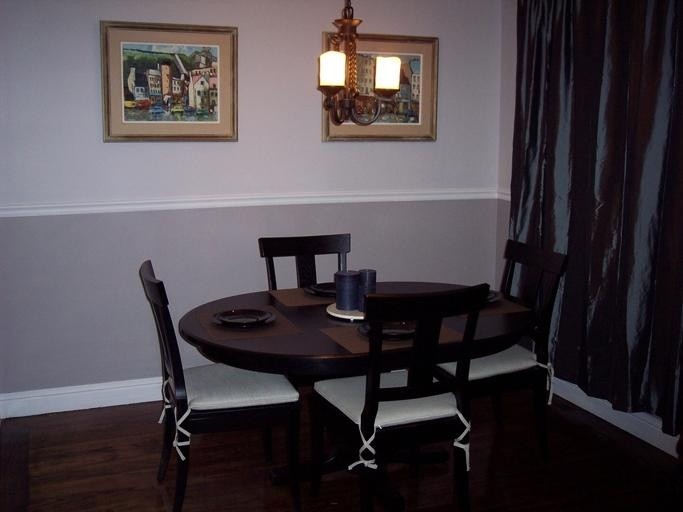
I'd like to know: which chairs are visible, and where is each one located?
[434,237,570,460]
[310,280,492,511]
[138,257,304,511]
[257,234,352,291]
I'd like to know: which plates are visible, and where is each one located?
[326,302,366,321]
[214,309,272,328]
[309,282,336,296]
[358,322,417,340]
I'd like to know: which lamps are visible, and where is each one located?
[316,0,402,126]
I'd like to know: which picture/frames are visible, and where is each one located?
[322,31,441,143]
[98,19,238,142]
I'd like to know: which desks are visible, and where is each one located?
[180,281,531,493]
